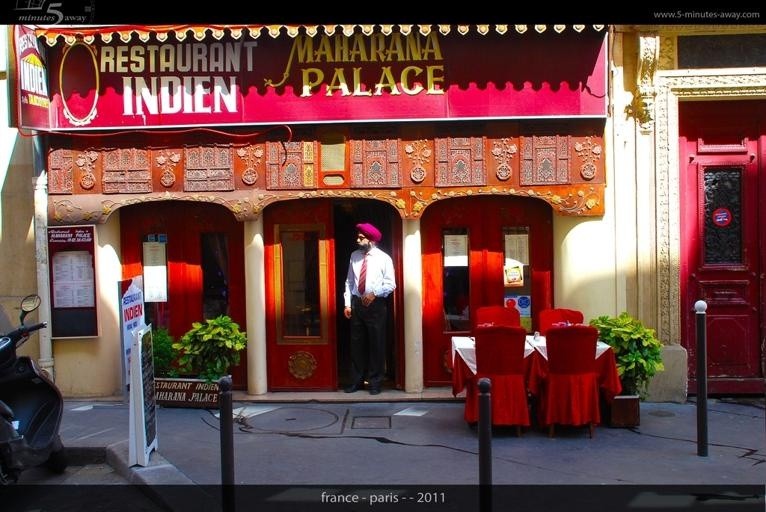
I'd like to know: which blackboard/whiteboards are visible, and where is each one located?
[138,323,159,456]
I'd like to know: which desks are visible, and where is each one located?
[449,334,613,427]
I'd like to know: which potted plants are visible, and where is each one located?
[151,316,247,411]
[591,314,665,428]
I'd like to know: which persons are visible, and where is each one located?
[343,222,397,395]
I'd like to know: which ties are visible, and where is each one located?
[358,253,367,295]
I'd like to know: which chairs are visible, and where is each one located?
[469,305,597,440]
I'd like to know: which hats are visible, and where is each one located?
[356,223,382,242]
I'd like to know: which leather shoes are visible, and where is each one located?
[369,384,382,395]
[345,381,363,393]
